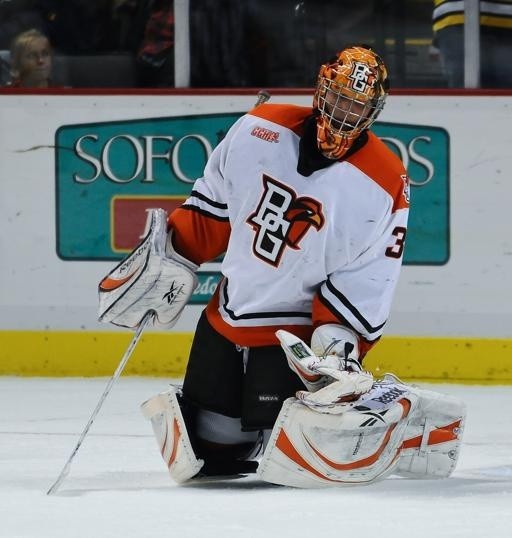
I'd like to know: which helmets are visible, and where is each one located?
[314,45,390,160]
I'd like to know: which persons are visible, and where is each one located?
[428,0,512,90]
[2,27,73,91]
[91,42,466,485]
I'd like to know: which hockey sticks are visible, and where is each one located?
[46,310,153,498]
